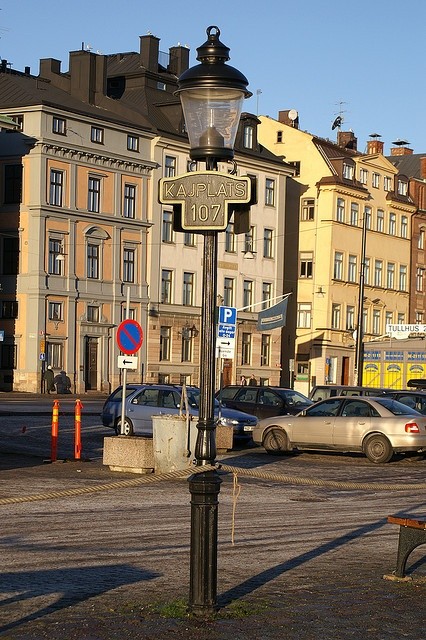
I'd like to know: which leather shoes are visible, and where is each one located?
[46,390,50,395]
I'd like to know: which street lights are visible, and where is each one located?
[156,24,257,615]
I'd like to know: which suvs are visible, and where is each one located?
[213,385,313,418]
[101,382,258,444]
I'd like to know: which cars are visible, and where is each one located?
[251,396,425,464]
[343,391,426,416]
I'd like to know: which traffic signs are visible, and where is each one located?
[216,305,236,359]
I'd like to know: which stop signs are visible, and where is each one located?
[116,320,143,354]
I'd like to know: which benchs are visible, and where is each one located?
[380,513,425,583]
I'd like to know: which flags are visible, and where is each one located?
[253,298,291,332]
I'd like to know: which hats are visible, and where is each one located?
[59,371,66,374]
[47,364,52,370]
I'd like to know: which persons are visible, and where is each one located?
[250,374,258,386]
[42,364,55,394]
[238,374,247,386]
[52,369,73,394]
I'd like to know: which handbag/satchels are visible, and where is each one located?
[65,390,71,394]
[50,386,55,392]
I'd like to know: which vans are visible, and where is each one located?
[309,385,387,403]
